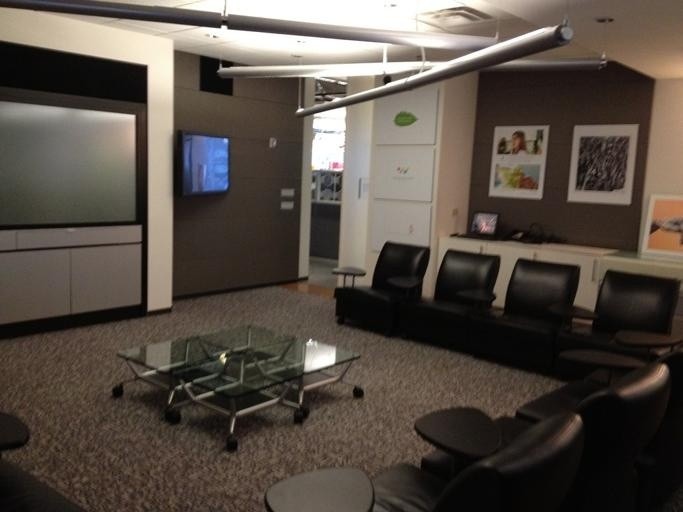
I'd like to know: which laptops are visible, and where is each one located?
[462,210,500,240]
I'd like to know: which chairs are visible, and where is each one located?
[457,256,580,374]
[331,241,431,340]
[514,350,682,467]
[548,268,681,384]
[414,405,585,512]
[264,459,457,510]
[1,408,89,511]
[388,247,500,349]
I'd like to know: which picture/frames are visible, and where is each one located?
[637,192,683,262]
[566,123,639,207]
[488,125,550,200]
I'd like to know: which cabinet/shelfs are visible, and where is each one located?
[0,221,144,342]
[438,237,683,323]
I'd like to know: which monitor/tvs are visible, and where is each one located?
[178,131,229,198]
[0,85,148,228]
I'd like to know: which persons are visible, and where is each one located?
[511,131,528,155]
